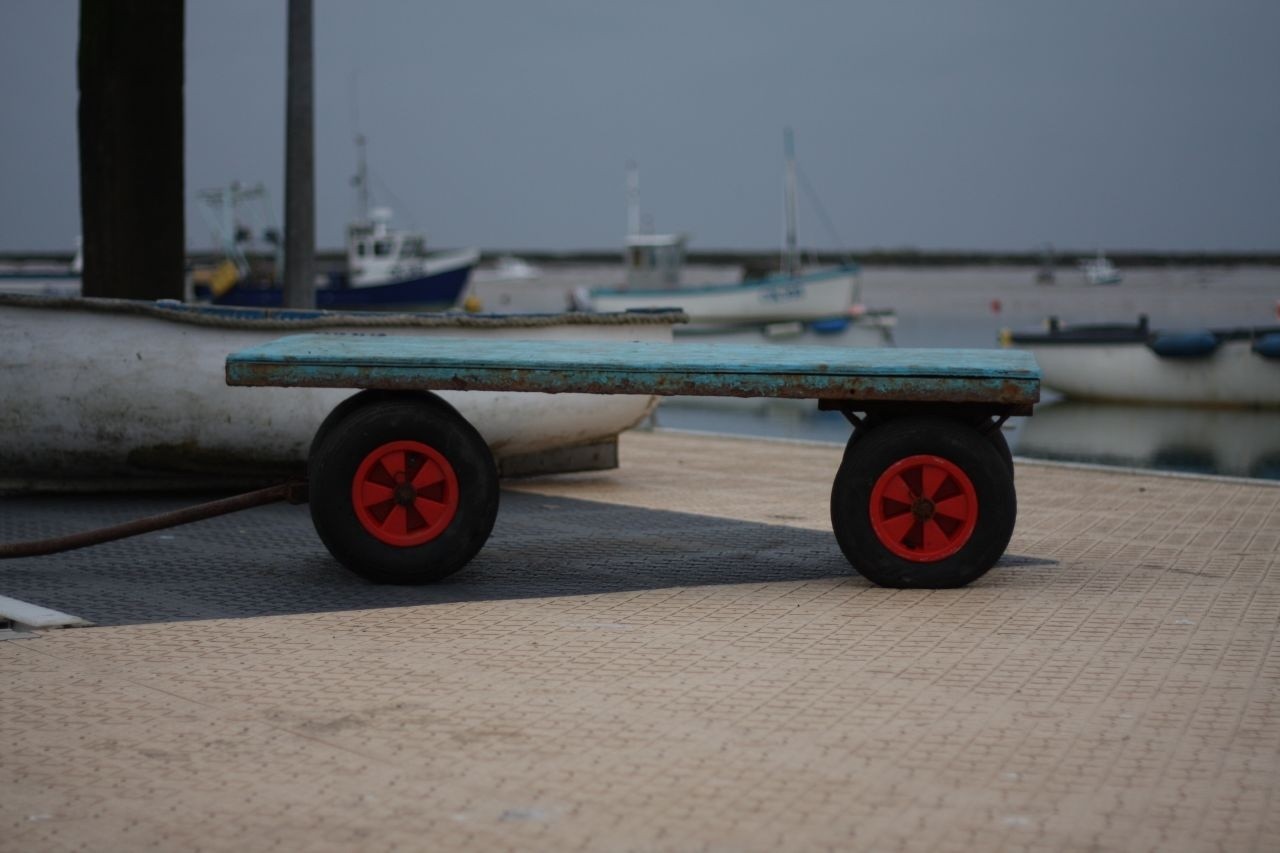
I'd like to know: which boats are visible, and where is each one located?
[181,122,485,316]
[997,310,1280,412]
[992,394,1278,484]
[563,125,867,336]
[2,280,694,483]
